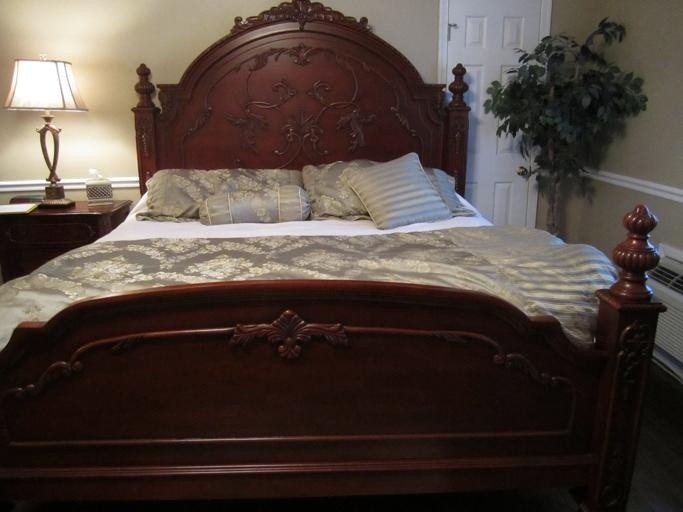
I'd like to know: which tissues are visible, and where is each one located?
[85,168,115,207]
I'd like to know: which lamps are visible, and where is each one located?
[3,59,91,210]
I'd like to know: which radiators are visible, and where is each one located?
[644,240,682,386]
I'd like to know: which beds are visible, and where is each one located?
[0,0,669,510]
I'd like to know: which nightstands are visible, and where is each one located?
[0,198,133,281]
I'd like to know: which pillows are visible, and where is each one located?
[298,156,476,223]
[340,149,454,232]
[197,184,311,227]
[134,161,303,223]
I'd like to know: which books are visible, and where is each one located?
[0,202,43,215]
